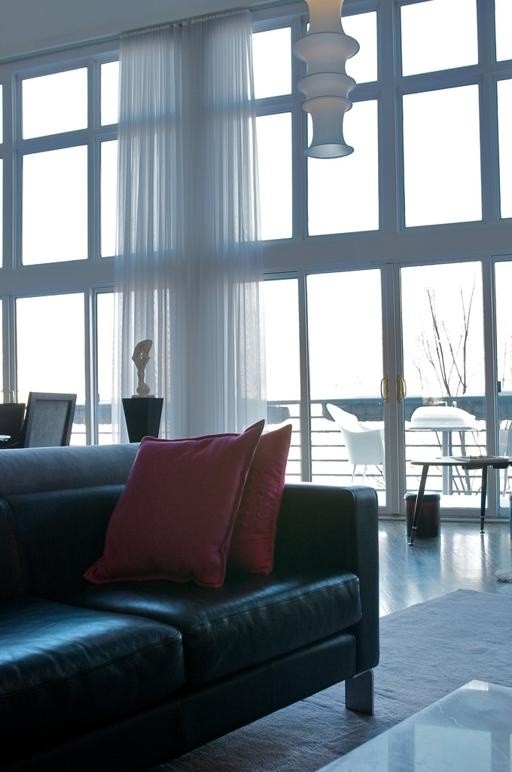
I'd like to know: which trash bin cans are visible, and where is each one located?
[404,493,440,538]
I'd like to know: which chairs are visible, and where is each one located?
[326,402,386,483]
[0,391,77,448]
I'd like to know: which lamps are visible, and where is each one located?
[292,1,361,161]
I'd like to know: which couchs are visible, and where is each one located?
[0,440,381,771]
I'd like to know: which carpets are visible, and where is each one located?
[152,587,511,772]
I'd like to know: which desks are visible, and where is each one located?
[404,455,509,547]
[406,425,474,496]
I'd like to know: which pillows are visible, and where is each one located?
[81,416,294,591]
